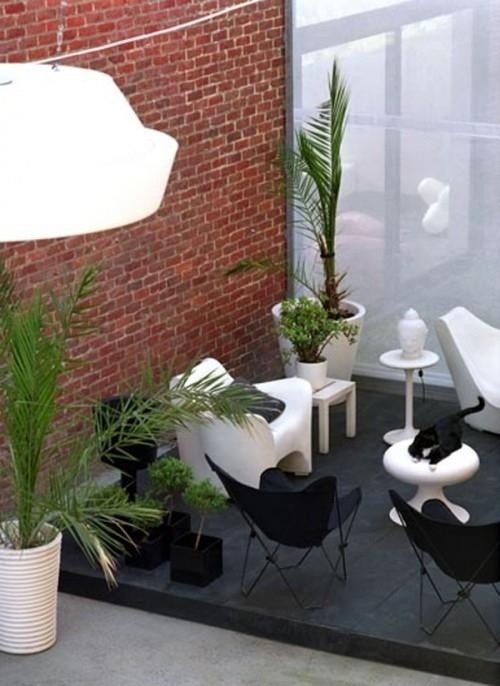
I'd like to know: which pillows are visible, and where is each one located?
[221,375,287,424]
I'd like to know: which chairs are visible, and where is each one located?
[388,487,499,647]
[203,453,363,610]
[169,357,313,500]
[435,305,500,434]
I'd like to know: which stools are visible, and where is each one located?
[290,374,358,455]
[382,438,480,529]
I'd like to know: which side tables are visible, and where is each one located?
[379,349,440,445]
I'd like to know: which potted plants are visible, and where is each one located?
[270,295,360,391]
[220,52,366,381]
[0,255,282,655]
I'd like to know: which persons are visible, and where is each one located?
[396,307,430,359]
